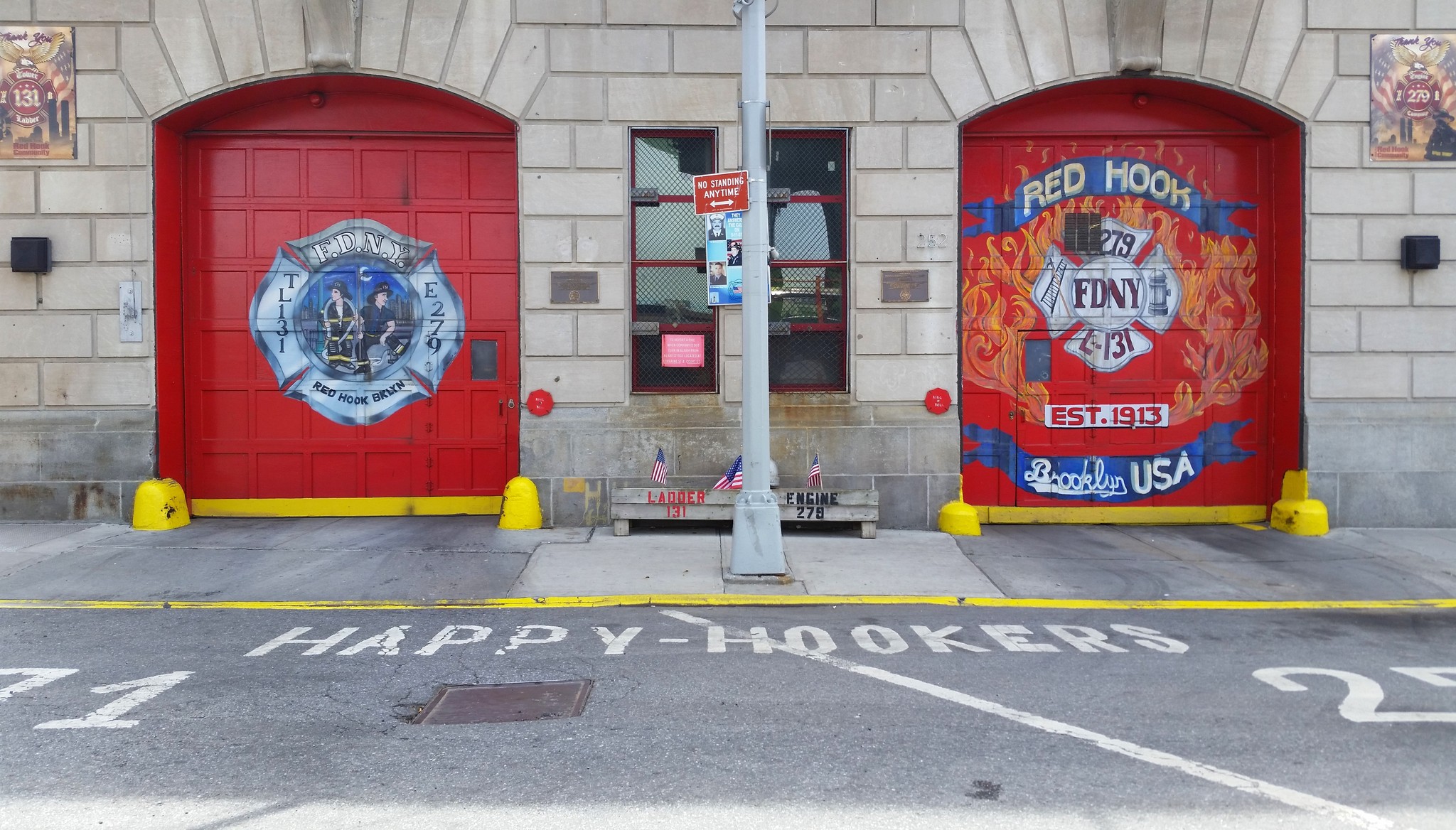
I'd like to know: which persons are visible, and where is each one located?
[710,263,727,285]
[728,242,742,266]
[708,214,726,241]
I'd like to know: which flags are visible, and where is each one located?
[805,453,821,490]
[651,447,667,485]
[712,455,742,489]
